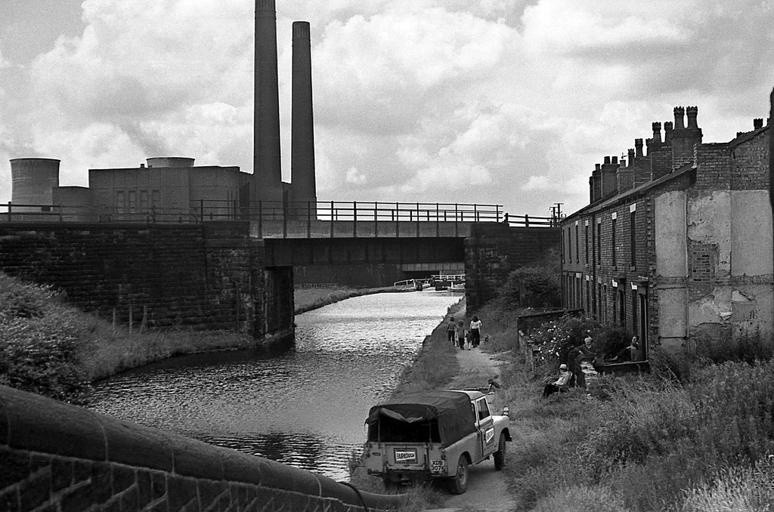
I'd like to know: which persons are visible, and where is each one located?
[455,320,465,349]
[628,334,641,361]
[572,335,603,366]
[465,329,472,350]
[539,363,571,399]
[445,316,457,345]
[469,315,482,348]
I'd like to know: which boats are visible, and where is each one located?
[390,273,466,293]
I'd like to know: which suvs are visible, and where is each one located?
[360,384,517,496]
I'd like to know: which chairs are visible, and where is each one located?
[554,371,573,396]
